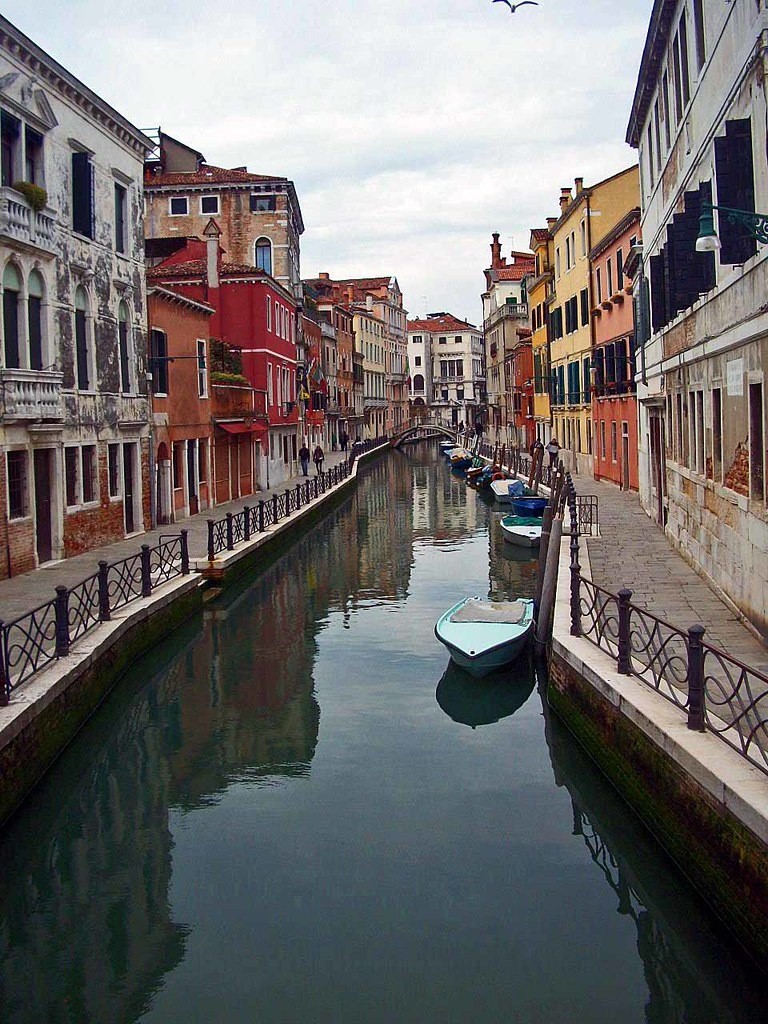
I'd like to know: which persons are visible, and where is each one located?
[313,444,324,475]
[332,431,371,452]
[529,438,544,465]
[298,443,310,477]
[442,418,483,439]
[545,438,562,468]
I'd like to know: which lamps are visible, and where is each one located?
[524,376,554,388]
[695,203,768,251]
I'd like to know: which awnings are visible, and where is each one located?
[218,423,268,443]
[363,400,389,412]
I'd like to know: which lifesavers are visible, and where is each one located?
[492,473,505,480]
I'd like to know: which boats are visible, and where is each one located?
[439,439,514,487]
[433,595,535,670]
[500,513,543,548]
[489,478,532,503]
[509,496,548,516]
